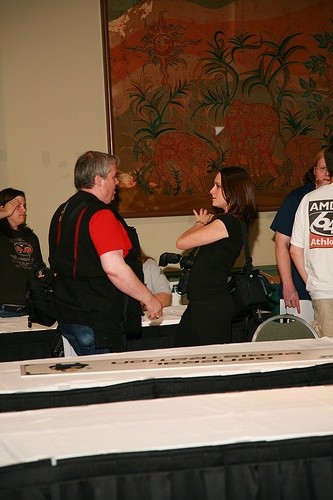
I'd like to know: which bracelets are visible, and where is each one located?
[195,221,207,227]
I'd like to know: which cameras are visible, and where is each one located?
[158,252,195,294]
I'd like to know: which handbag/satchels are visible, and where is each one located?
[27,263,55,328]
[228,270,276,311]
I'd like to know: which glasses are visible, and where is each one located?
[316,166,328,173]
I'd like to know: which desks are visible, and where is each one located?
[0,385,333,500]
[0,305,189,363]
[0,336,333,412]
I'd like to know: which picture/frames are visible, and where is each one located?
[99,0,333,219]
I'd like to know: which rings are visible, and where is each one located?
[155,316,159,320]
[288,301,292,304]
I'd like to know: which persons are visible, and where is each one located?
[270,146,333,338]
[173,167,259,346]
[50,151,173,357]
[0,188,45,362]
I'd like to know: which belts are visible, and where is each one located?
[1,306,27,314]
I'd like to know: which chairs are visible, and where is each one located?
[251,313,319,342]
